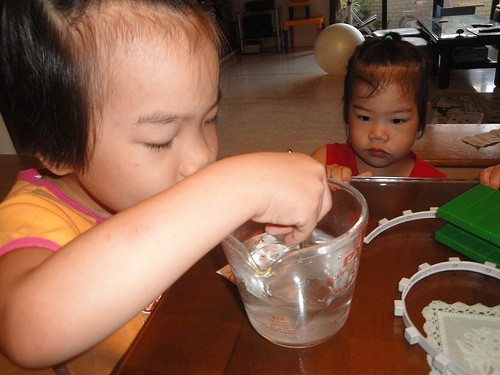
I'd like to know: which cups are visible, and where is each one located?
[221,178,369,348]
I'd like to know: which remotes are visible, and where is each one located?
[479,28,500,33]
[471,23,493,27]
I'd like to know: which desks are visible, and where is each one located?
[417,14,500,89]
[110,176,500,375]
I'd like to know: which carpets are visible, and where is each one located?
[428,93,500,124]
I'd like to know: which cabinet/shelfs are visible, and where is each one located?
[236,8,282,55]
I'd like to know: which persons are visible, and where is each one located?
[309,31,500,191]
[0,0,334,375]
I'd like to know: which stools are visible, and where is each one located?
[283,0,325,54]
[373,27,420,37]
[402,37,428,48]
